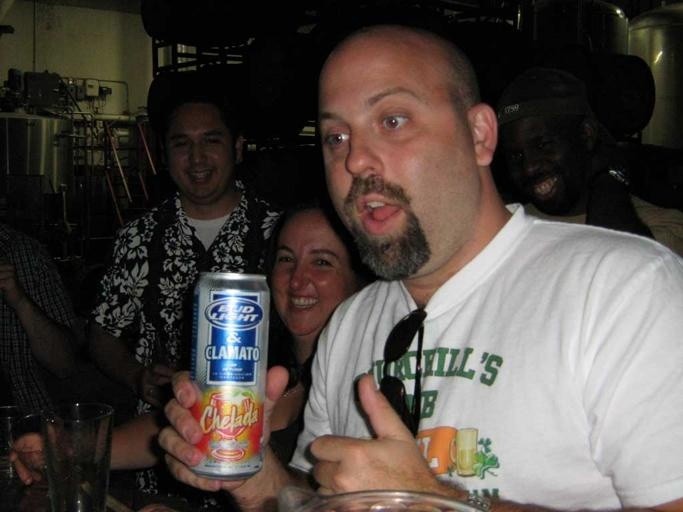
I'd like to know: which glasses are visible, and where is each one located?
[380,307,427,438]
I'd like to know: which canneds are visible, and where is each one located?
[188,270,270,482]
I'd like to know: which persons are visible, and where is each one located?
[0,222,83,436]
[9,196,377,512]
[493,67,682,260]
[89,89,297,512]
[158,21,682,512]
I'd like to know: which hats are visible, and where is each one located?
[497,68,586,126]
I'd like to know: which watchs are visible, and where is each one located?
[588,163,629,190]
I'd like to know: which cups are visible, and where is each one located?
[278,485,483,512]
[42,404,114,512]
[8,413,88,505]
[1,406,17,478]
